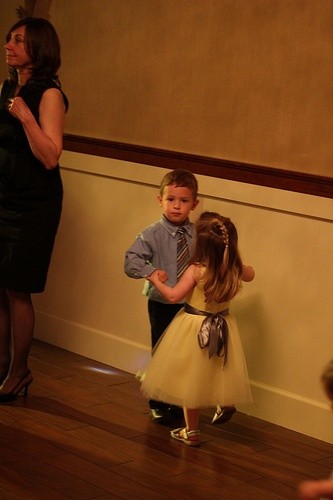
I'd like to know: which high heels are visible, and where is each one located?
[0,369,33,402]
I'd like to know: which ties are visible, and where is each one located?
[176,227,191,284]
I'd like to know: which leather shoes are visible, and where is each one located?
[147,398,176,424]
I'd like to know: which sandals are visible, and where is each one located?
[170,427,201,446]
[211,402,236,424]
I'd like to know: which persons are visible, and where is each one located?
[0,16,68,403]
[124,170,203,421]
[145,212,254,446]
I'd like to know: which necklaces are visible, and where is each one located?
[15,82,24,89]
